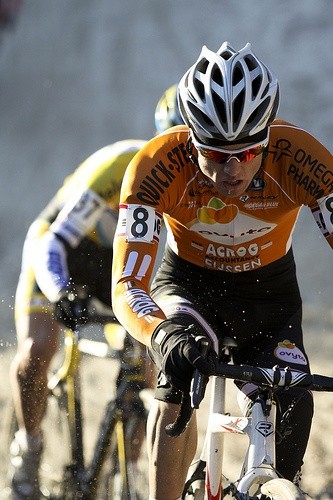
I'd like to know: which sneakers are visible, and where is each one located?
[12,430,44,500]
[114,470,137,500]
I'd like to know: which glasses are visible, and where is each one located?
[189,126,270,165]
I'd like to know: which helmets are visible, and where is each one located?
[175,41,281,142]
[154,85,185,133]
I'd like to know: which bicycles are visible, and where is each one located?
[8,289,153,500]
[160,337,333,500]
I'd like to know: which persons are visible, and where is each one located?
[7,83,187,500]
[111,40,333,500]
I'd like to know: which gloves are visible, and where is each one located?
[54,294,89,329]
[151,319,213,389]
